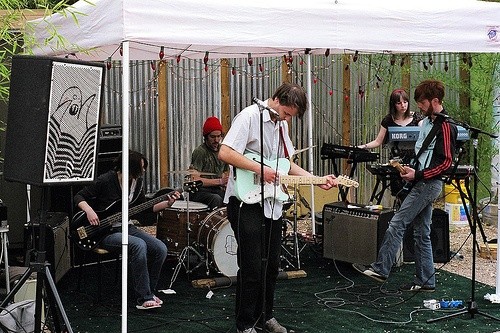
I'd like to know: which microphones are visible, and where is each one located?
[251,96,280,116]
[434,111,449,118]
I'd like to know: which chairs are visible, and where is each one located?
[73,201,136,304]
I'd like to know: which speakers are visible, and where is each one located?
[25,211,71,283]
[3,55,107,186]
[321,202,394,264]
[70,136,122,211]
[403,209,451,263]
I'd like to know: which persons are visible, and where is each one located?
[217,81,339,333]
[73,149,182,310]
[188,117,230,212]
[350,79,459,293]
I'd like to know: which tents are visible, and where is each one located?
[24,0,500,333]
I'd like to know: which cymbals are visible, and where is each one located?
[167,170,216,176]
[295,145,318,155]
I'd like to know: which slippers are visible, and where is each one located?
[153,295,163,305]
[136,301,161,309]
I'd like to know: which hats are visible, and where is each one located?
[203,117,222,135]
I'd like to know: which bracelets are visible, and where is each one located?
[220,179,223,185]
[168,202,172,206]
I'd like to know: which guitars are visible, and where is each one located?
[70,179,204,249]
[389,157,419,190]
[231,153,360,205]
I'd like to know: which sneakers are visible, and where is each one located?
[237,327,257,333]
[399,282,436,292]
[255,317,288,333]
[352,263,387,282]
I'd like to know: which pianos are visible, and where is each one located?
[366,162,496,263]
[320,143,380,204]
[382,125,470,149]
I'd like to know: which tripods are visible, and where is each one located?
[167,192,215,289]
[0,186,74,333]
[427,117,500,325]
[280,184,310,271]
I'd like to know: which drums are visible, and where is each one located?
[156,199,211,256]
[196,207,241,278]
[281,185,302,221]
